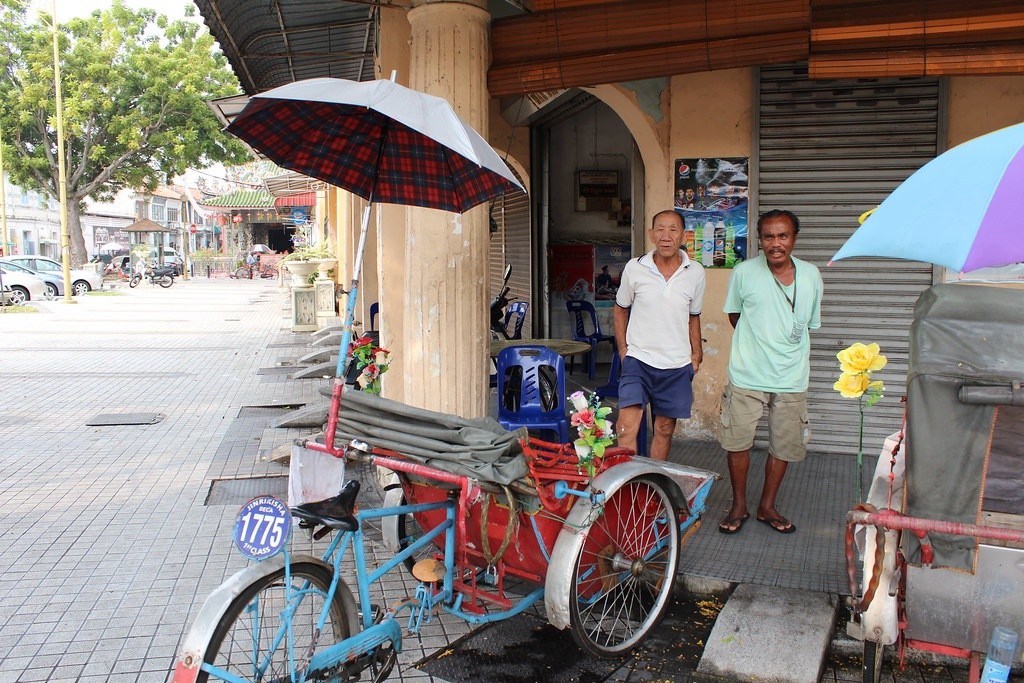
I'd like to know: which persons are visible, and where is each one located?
[675,181,748,210]
[719,209,824,533]
[92,256,106,283]
[247,252,258,270]
[613,210,705,461]
[597,266,612,293]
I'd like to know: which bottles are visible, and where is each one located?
[979,626,1018,683]
[682,217,735,267]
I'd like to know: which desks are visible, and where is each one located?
[490,339,592,450]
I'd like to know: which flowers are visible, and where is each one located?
[832,341,887,505]
[567,392,624,489]
[349,337,394,396]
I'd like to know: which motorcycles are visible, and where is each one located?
[129,259,178,289]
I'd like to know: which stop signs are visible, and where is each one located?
[189,224,197,234]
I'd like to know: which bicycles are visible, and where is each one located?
[235,259,279,281]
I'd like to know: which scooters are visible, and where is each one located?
[345,264,558,414]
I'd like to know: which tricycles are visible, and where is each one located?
[172,428,724,683]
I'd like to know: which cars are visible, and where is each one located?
[103,256,130,276]
[0,259,74,298]
[0,255,104,296]
[147,247,184,277]
[0,267,50,307]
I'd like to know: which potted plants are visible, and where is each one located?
[278,241,338,287]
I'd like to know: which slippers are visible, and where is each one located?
[719,510,751,534]
[757,512,796,534]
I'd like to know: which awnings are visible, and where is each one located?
[263,173,327,198]
[192,0,376,97]
[274,192,317,217]
[205,93,269,160]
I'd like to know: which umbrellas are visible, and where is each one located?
[102,242,122,251]
[249,245,270,252]
[826,123,1024,280]
[222,70,526,449]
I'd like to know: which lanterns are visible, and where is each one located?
[233,214,242,225]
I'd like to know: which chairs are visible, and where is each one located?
[594,353,655,457]
[496,345,570,462]
[565,300,616,381]
